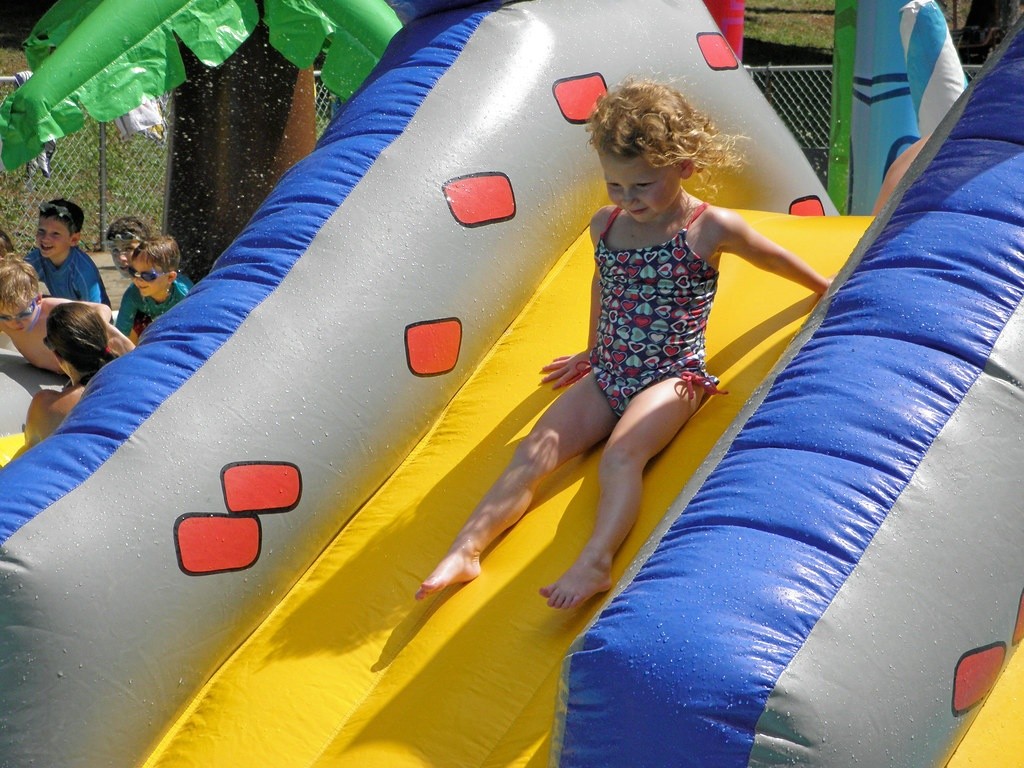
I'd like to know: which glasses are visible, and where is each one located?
[109,246,136,255]
[39,201,77,233]
[1,297,37,323]
[126,265,169,282]
[42,336,61,355]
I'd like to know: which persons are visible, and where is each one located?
[115,235,195,337]
[0,229,15,262]
[22,198,115,326]
[0,252,136,375]
[415,77,833,611]
[9,302,120,463]
[106,215,157,339]
[871,132,934,217]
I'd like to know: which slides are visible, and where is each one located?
[137,199,884,768]
